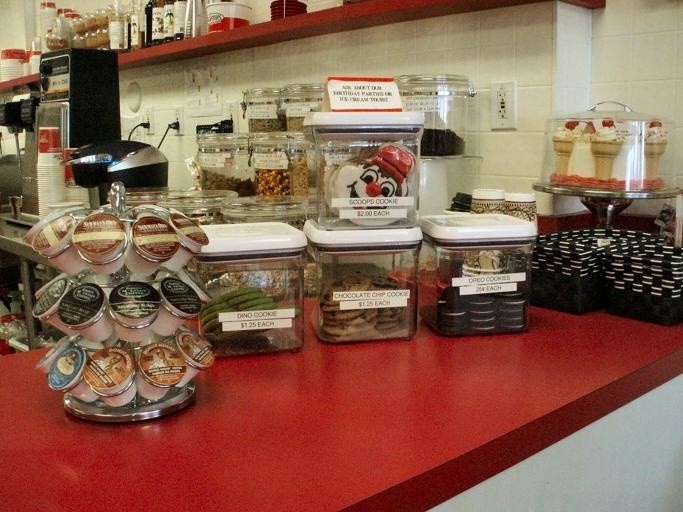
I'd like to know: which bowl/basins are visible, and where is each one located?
[206,2,252,34]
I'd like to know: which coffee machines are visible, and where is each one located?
[71,138,168,205]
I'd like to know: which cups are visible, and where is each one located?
[152,277,202,336]
[109,281,161,343]
[161,213,209,273]
[84,348,137,408]
[462,188,502,282]
[125,217,180,277]
[64,147,90,207]
[504,193,537,236]
[0,49,25,83]
[175,330,215,389]
[36,335,68,374]
[135,343,187,402]
[48,347,100,404]
[32,215,84,276]
[36,127,65,221]
[59,283,113,343]
[74,213,127,275]
[32,278,75,337]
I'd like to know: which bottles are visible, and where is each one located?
[245,87,284,132]
[123,0,130,50]
[145,0,164,46]
[130,0,142,50]
[221,198,319,231]
[282,84,324,132]
[162,0,173,42]
[125,186,190,210]
[173,0,186,40]
[249,132,310,206]
[157,189,239,226]
[197,132,252,197]
[395,74,477,155]
[184,0,204,39]
[109,0,125,51]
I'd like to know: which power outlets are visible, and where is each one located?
[173,106,184,135]
[489,81,517,129]
[144,112,154,136]
[226,101,238,133]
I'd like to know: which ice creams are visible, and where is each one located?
[579,121,596,175]
[590,119,622,180]
[645,120,668,180]
[553,121,579,175]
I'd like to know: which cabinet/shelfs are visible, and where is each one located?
[1,0,606,92]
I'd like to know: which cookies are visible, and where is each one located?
[201,286,303,338]
[467,293,496,332]
[501,289,526,329]
[375,275,403,330]
[320,273,379,338]
[436,286,467,333]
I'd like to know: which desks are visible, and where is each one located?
[1,266,683,512]
